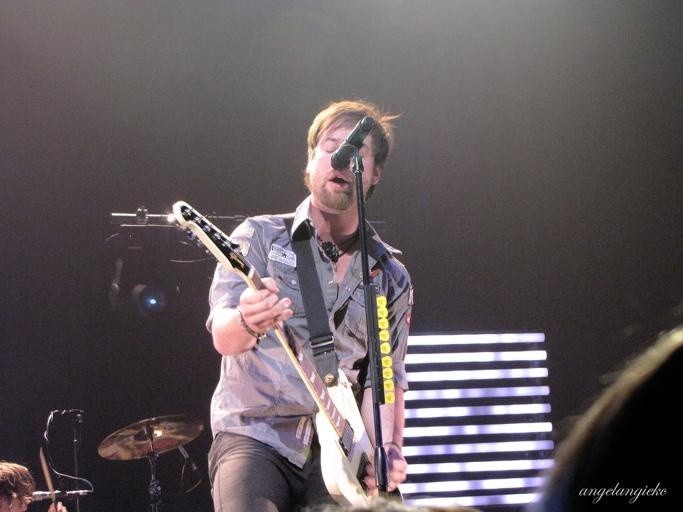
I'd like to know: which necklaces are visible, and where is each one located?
[315,231,360,264]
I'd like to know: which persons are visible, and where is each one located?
[522,323,681,509]
[0,459,68,512]
[205,97,417,512]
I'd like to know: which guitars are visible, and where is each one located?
[166,200,404,511]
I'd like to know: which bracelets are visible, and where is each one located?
[235,303,268,340]
[382,439,404,451]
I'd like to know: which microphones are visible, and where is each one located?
[31,489,93,501]
[330,115,377,171]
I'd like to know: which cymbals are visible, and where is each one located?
[98,414,204,461]
